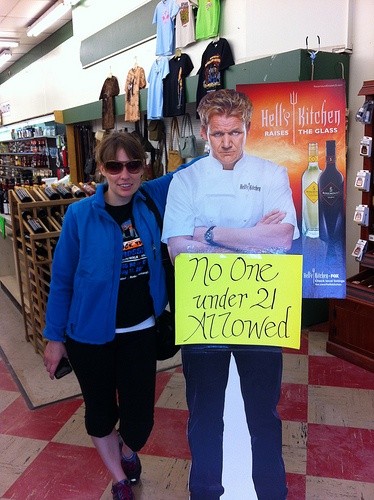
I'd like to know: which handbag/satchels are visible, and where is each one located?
[179,113,198,159]
[167,151,185,172]
[147,120,165,141]
[155,309,182,361]
[140,137,155,169]
[84,157,96,175]
[153,161,164,178]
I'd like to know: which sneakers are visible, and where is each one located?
[117,428,142,484]
[111,479,135,500]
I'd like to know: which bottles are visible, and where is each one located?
[41,184,60,200]
[91,182,99,188]
[26,214,42,233]
[67,181,85,198]
[0,167,51,185]
[7,139,46,152]
[318,139,343,243]
[50,238,56,244]
[55,211,63,225]
[13,186,32,203]
[79,181,95,197]
[302,142,322,239]
[24,232,44,260]
[51,183,72,199]
[0,155,47,167]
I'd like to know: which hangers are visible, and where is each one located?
[100,34,221,79]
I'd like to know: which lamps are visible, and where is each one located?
[0,50,12,67]
[0,39,20,48]
[26,0,80,37]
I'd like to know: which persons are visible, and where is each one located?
[42,132,208,500]
[163,89,294,500]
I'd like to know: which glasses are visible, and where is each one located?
[101,159,144,175]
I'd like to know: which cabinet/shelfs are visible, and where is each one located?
[0,136,57,179]
